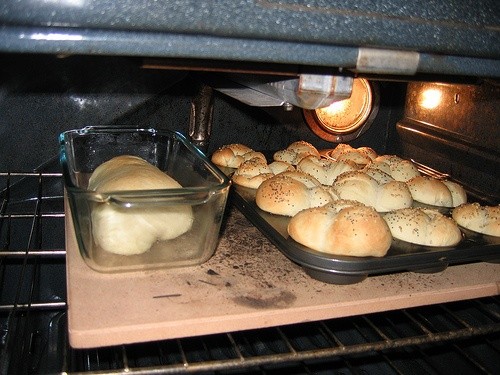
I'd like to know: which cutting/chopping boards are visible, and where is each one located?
[63,170,500,349]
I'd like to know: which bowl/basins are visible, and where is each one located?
[58,125,232,272]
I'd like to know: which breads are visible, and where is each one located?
[87,155,194,255]
[212,141,500,256]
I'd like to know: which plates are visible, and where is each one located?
[211,148,500,284]
